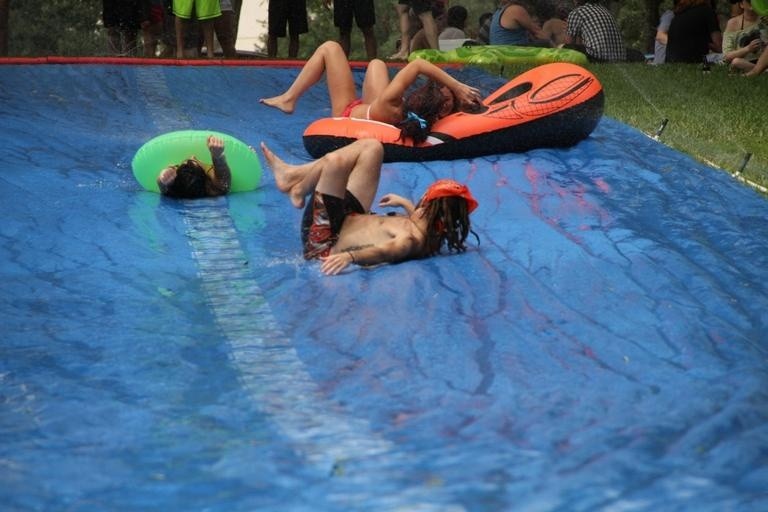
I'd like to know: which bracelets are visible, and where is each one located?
[346,250,357,264]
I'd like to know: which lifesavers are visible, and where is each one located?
[132,131,262,194]
[304,62,605,159]
[407,44,587,64]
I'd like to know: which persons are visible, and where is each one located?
[333,2,377,62]
[639,1,766,79]
[155,133,233,200]
[258,136,480,281]
[268,1,308,60]
[257,37,483,150]
[409,77,481,124]
[388,2,627,64]
[101,1,243,60]
